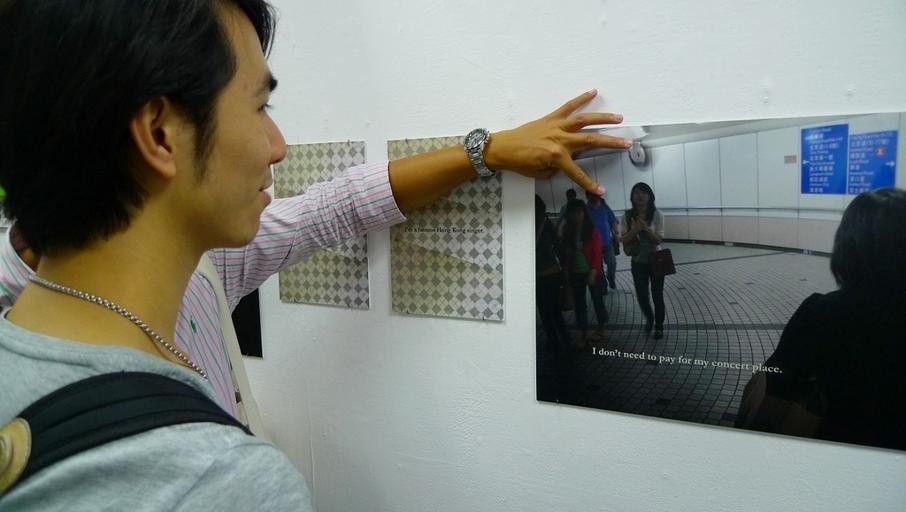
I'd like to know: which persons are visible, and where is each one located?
[554,188,579,233]
[731,182,906,454]
[536,192,574,350]
[556,199,611,344]
[618,181,668,339]
[582,187,622,296]
[0,86,639,439]
[0,0,323,512]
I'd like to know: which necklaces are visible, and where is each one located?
[25,271,212,384]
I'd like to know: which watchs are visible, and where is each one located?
[462,127,497,178]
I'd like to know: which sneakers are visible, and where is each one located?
[607,276,616,289]
[591,328,605,341]
[575,334,589,349]
[645,316,654,330]
[654,325,664,339]
[602,286,608,295]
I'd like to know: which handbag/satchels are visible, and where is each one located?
[646,243,676,281]
[611,229,621,256]
